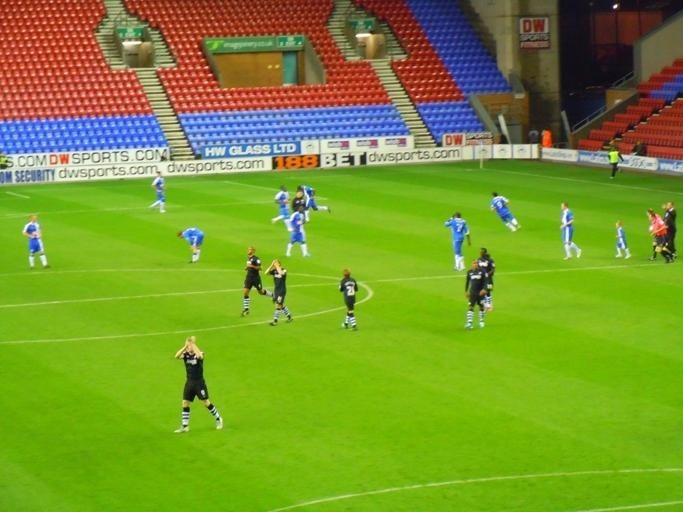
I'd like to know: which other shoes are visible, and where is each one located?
[576,248,583,258]
[512,228,516,232]
[624,253,632,259]
[616,253,623,258]
[517,224,521,230]
[609,176,614,180]
[563,254,573,261]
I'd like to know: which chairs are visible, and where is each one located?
[576,54,681,163]
[0,1,177,162]
[120,0,418,150]
[349,1,517,147]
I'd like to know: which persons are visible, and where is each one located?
[149,170,169,214]
[490,192,522,232]
[645,201,677,263]
[174,336,223,434]
[615,219,631,259]
[271,186,330,257]
[464,247,496,328]
[240,246,360,330]
[560,201,582,260]
[444,212,471,272]
[608,146,624,179]
[23,214,50,270]
[178,227,204,263]
[634,141,644,155]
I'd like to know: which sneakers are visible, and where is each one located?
[214,417,224,432]
[240,308,250,318]
[173,425,189,434]
[269,320,278,327]
[479,319,486,329]
[341,323,358,331]
[285,316,294,323]
[464,323,474,330]
